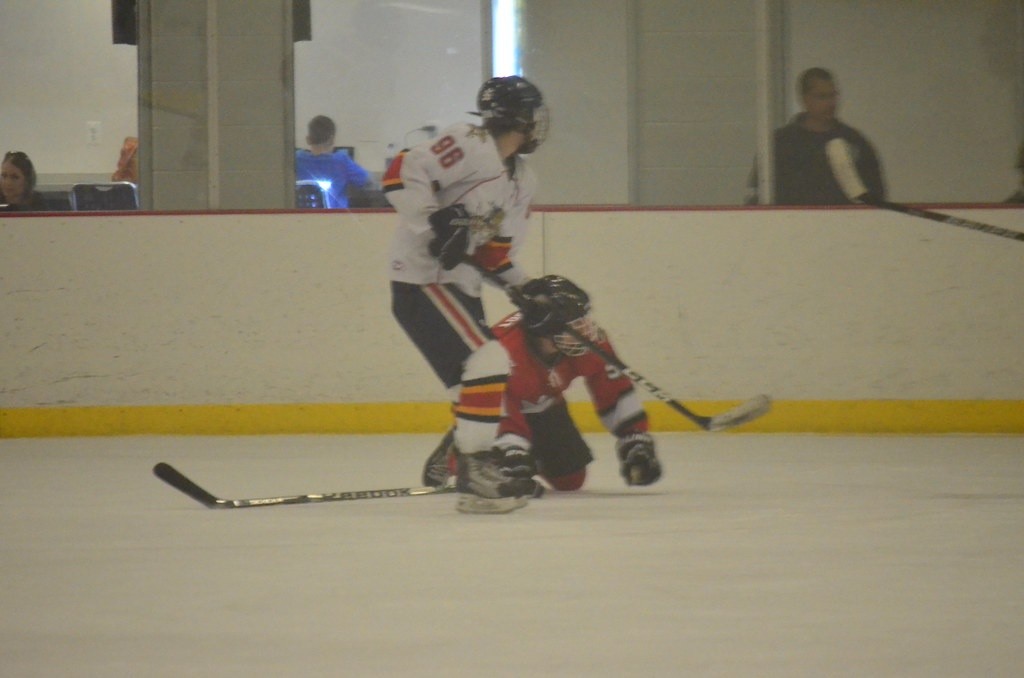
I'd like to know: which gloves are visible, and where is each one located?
[616,435,660,485]
[426,204,469,269]
[500,449,543,498]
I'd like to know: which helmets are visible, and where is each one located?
[477,77,549,155]
[521,274,595,356]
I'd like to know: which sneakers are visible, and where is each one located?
[456,446,527,515]
[422,425,459,489]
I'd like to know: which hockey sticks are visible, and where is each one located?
[460,253,775,436]
[153,462,463,515]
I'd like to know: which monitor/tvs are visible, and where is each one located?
[331,146,354,160]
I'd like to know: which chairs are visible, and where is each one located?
[71,181,140,211]
[296,180,330,208]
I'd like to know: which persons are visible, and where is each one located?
[423,275,662,492]
[382,75,549,512]
[0,150,37,210]
[745,69,885,206]
[293,114,376,211]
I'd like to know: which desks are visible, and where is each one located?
[34,173,113,192]
[346,171,389,198]
